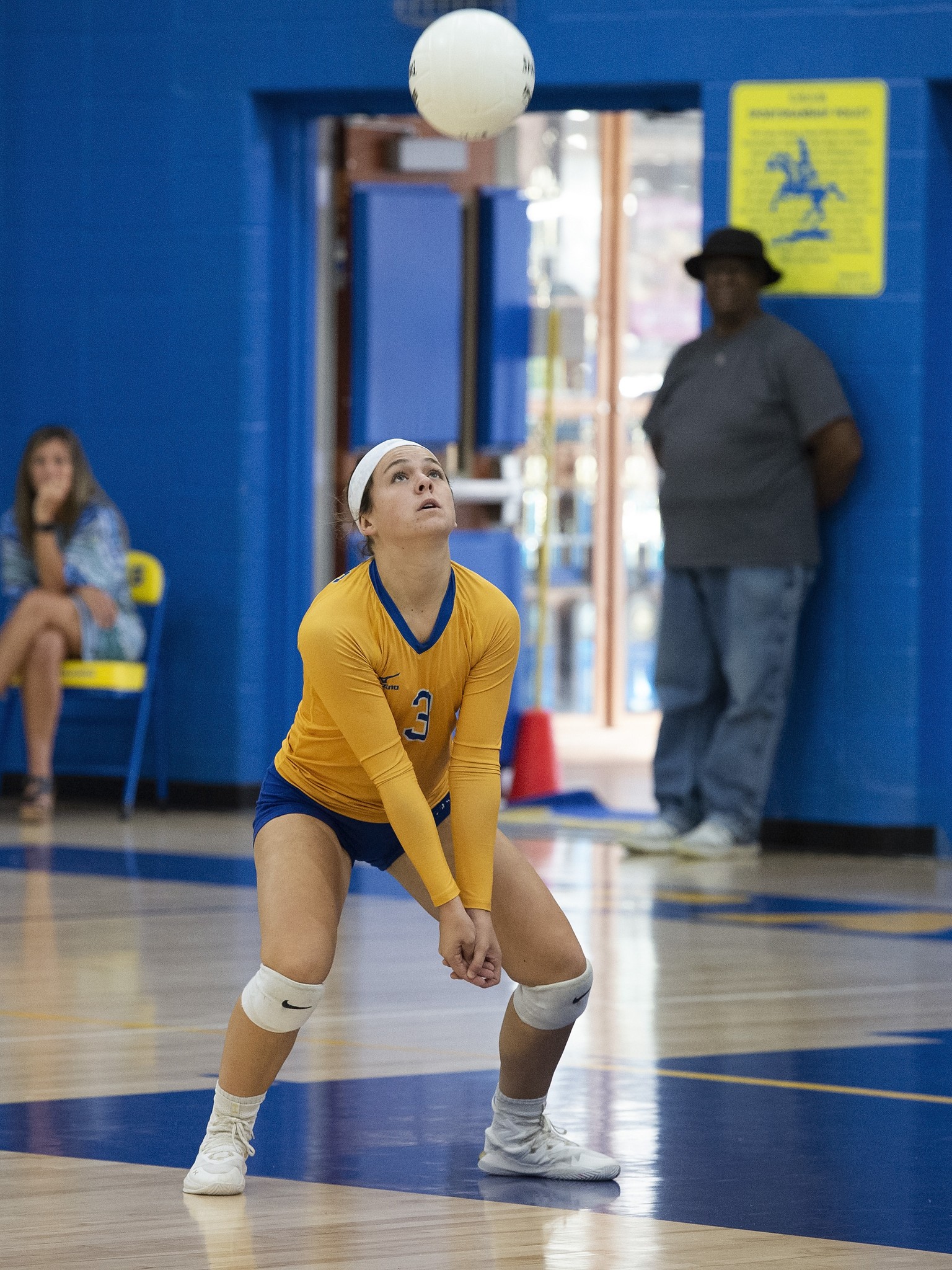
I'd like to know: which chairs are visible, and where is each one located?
[7,550,167,822]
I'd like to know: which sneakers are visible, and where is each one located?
[477,1115,621,1181]
[182,1108,258,1196]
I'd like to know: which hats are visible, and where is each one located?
[685,230,782,286]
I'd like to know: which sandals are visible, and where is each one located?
[17,776,55,820]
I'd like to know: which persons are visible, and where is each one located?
[619,228,863,859]
[183,438,620,1195]
[0,422,147,820]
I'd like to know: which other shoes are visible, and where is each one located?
[621,819,685,853]
[669,814,763,859]
